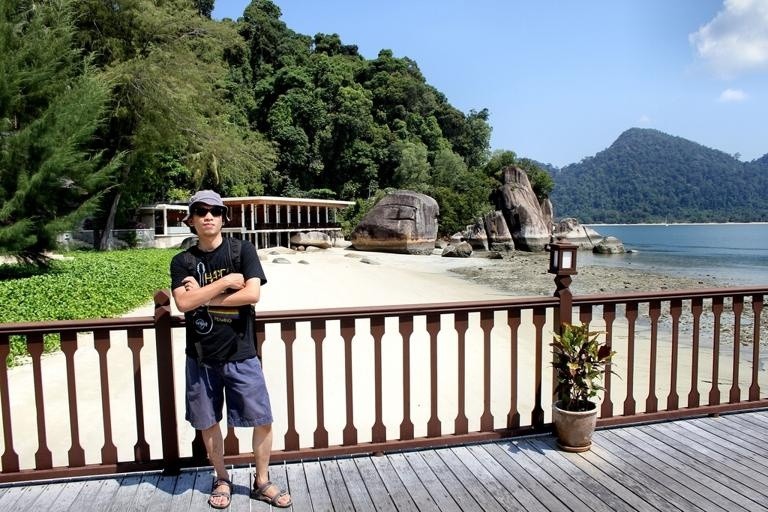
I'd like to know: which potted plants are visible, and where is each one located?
[548,321,623,452]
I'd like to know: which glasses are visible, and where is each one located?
[194,207,223,218]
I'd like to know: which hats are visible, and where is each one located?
[183,190,231,234]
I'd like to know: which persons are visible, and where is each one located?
[167,186,295,510]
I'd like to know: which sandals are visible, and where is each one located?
[252,475,293,507]
[207,479,233,507]
